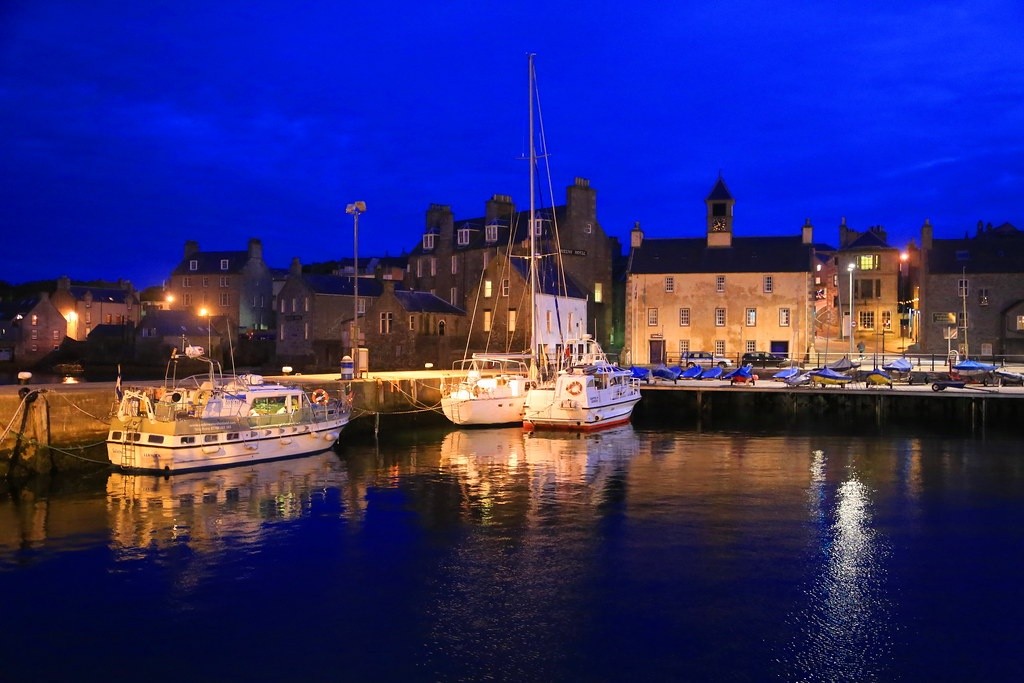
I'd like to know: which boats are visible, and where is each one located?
[808,368,853,384]
[772,369,811,387]
[523,337,643,431]
[652,364,681,381]
[951,361,1000,375]
[702,366,722,382]
[730,367,753,383]
[993,369,1023,383]
[865,368,891,385]
[627,365,649,380]
[680,365,703,380]
[105,345,349,475]
[725,366,751,380]
[889,360,913,375]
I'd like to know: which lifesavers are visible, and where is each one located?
[568,380,584,396]
[18,387,38,402]
[312,388,329,406]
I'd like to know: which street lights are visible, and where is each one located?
[899,253,909,360]
[847,263,858,361]
[346,202,368,377]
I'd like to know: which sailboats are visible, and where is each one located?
[440,53,563,426]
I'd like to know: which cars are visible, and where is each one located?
[742,350,791,368]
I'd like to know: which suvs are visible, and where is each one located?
[680,351,732,370]
